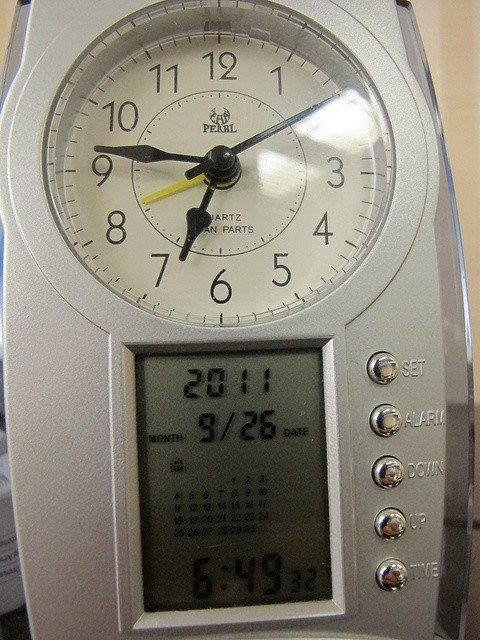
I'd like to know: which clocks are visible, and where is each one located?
[4,0,432,345]
